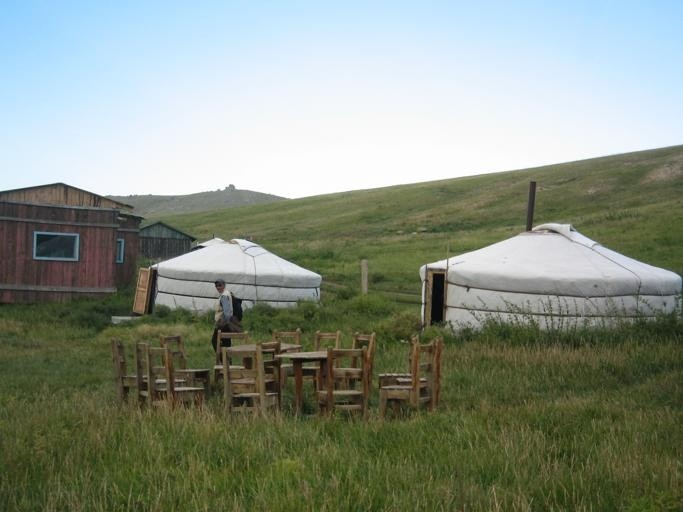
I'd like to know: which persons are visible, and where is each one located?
[212,279,242,366]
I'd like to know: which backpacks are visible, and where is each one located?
[230,292,243,322]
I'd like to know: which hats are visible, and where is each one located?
[215,280,225,285]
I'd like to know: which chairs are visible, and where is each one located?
[108,327,441,422]
